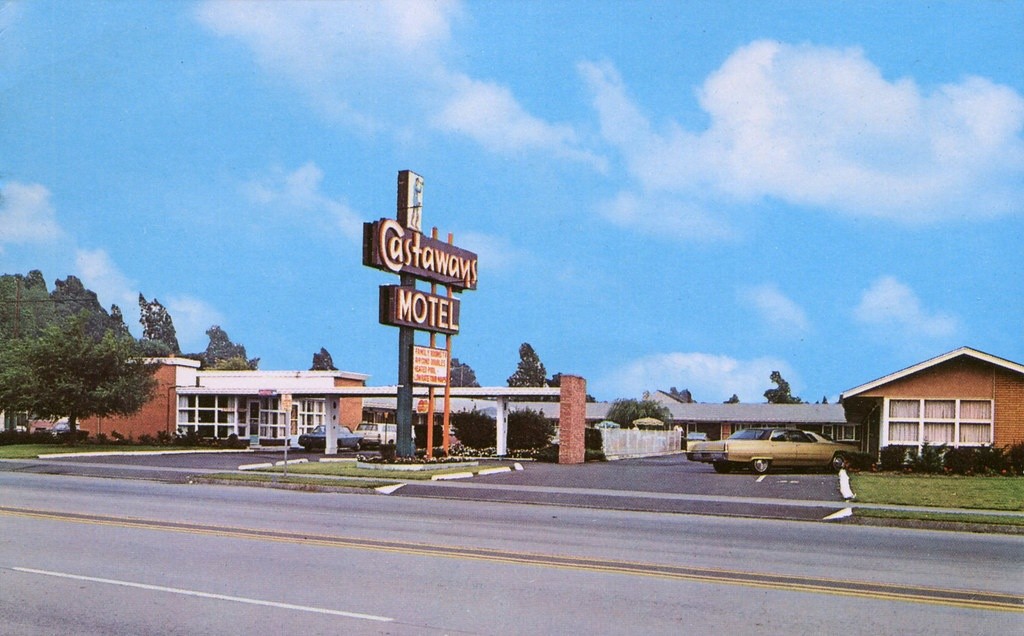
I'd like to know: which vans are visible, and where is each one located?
[353,422,416,447]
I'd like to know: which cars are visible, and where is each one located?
[682,427,862,474]
[802,431,839,444]
[49,420,82,437]
[297,424,364,452]
